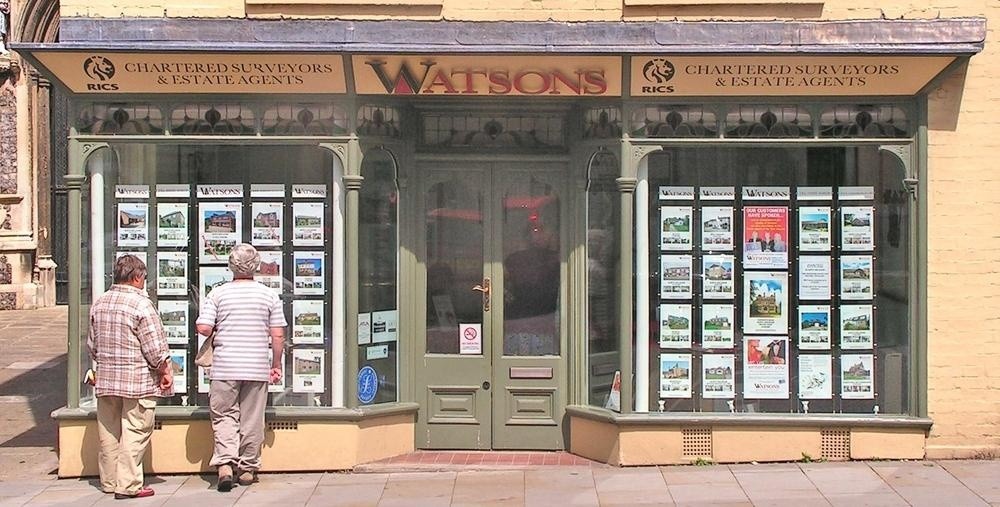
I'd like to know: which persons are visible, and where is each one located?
[767,339,785,365]
[194,244,289,492]
[749,231,786,252]
[86,254,174,499]
[748,339,764,365]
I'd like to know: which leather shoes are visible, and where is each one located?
[217,464,234,490]
[114,486,154,499]
[238,471,259,485]
